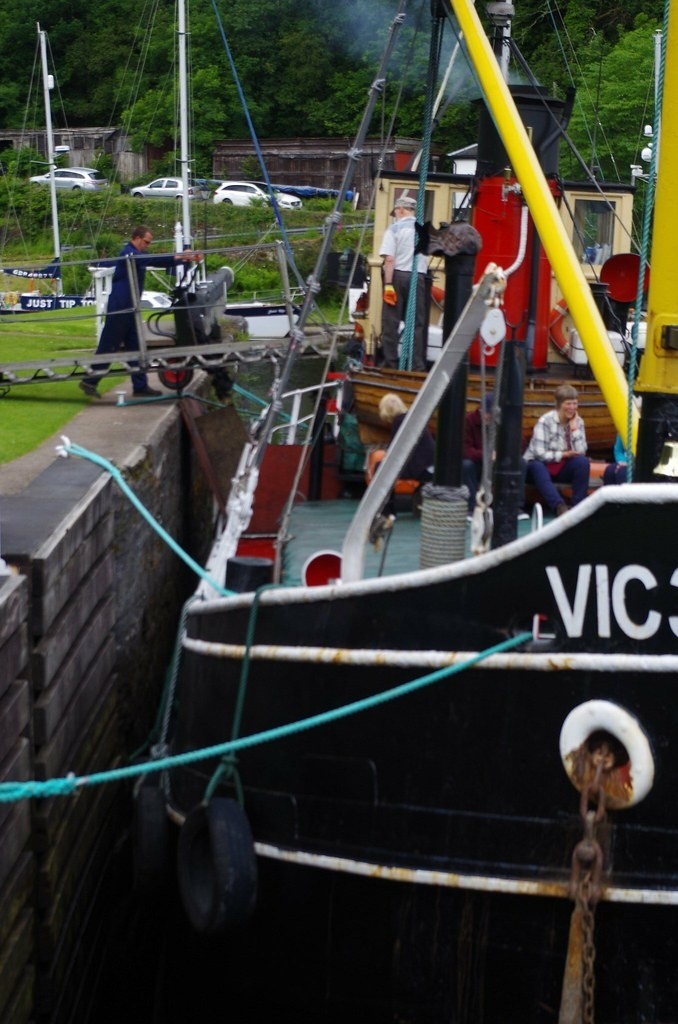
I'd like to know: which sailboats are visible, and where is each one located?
[0,0,355,344]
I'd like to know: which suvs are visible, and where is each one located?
[29,167,109,190]
[213,181,302,210]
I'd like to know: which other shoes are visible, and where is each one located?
[79,381,101,399]
[132,384,161,397]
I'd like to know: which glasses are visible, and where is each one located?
[142,238,150,244]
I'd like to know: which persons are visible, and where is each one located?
[79,226,164,400]
[463,393,497,511]
[522,383,590,516]
[381,197,427,372]
[367,392,437,546]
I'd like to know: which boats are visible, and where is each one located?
[136,0,678,1023]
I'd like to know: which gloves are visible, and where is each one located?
[384,283,397,306]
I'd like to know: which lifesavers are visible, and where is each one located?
[549,298,570,359]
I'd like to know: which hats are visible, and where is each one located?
[480,392,494,411]
[389,197,418,217]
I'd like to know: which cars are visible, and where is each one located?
[131,177,204,201]
